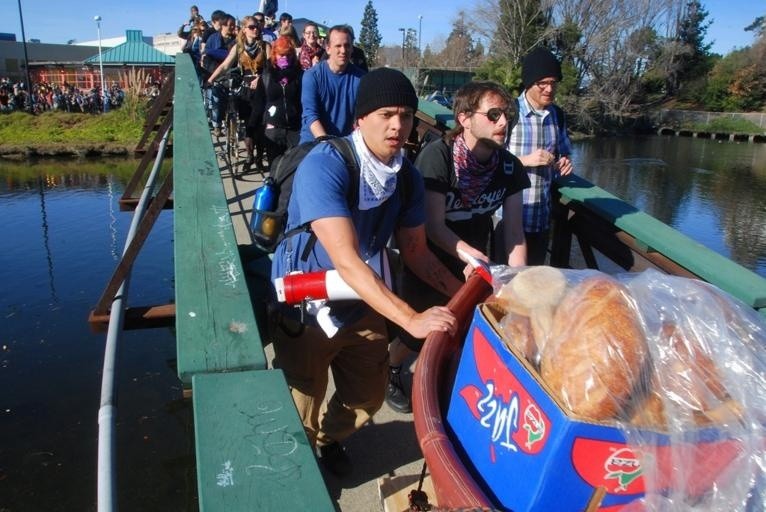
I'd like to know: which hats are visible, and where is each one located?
[355,67,417,119]
[317,27,326,37]
[522,47,563,90]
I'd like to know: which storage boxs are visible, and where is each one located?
[444,293,745,510]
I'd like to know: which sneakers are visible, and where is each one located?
[386,351,411,413]
[318,440,352,477]
[255,159,266,173]
[242,155,254,172]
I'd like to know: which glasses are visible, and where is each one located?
[534,81,560,89]
[244,24,259,30]
[464,107,517,123]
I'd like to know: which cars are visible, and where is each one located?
[427,93,449,110]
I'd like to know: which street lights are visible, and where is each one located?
[418,15,423,69]
[398,28,405,73]
[94,16,108,114]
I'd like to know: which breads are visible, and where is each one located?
[499,266,745,432]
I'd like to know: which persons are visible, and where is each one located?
[1,77,161,114]
[178,5,370,177]
[489,45,573,265]
[385,82,531,413]
[272,68,465,476]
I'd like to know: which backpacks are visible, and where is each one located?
[249,135,414,254]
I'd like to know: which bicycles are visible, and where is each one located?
[200,75,258,179]
